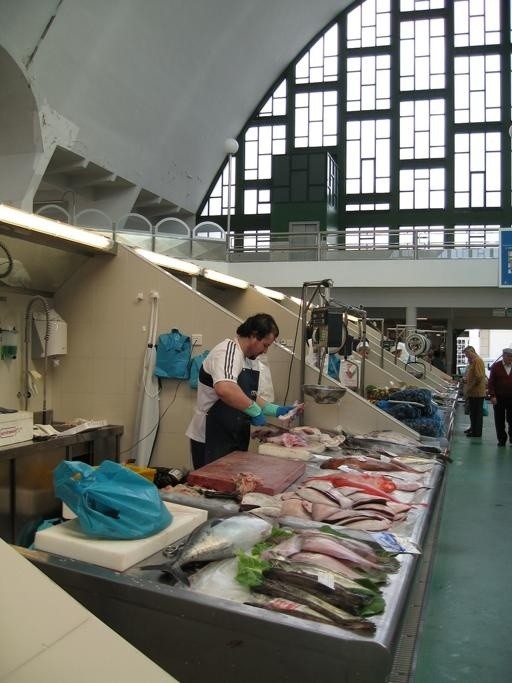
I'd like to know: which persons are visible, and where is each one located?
[187,313,304,475]
[390,345,402,358]
[488,348,512,447]
[464,346,486,437]
[357,341,369,358]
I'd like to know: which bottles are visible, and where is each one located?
[1,296,18,359]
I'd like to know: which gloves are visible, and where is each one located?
[264,401,301,422]
[244,401,268,426]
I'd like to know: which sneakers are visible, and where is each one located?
[464,428,512,447]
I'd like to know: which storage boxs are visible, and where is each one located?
[0,407,34,446]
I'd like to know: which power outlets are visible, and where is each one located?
[191,334,202,346]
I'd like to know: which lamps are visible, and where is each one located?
[222,138,240,262]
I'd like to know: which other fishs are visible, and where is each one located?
[302,458,441,507]
[139,511,272,588]
[339,428,452,465]
[240,428,453,634]
[262,531,398,634]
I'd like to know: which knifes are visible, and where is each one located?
[264,421,291,434]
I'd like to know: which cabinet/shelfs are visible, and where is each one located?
[0,420,123,545]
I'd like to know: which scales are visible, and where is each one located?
[404,333,433,380]
[299,305,348,404]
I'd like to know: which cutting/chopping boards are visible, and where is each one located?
[36,498,209,572]
[258,440,326,460]
[187,449,307,495]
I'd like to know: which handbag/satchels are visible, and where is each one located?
[465,398,489,416]
[52,458,173,541]
